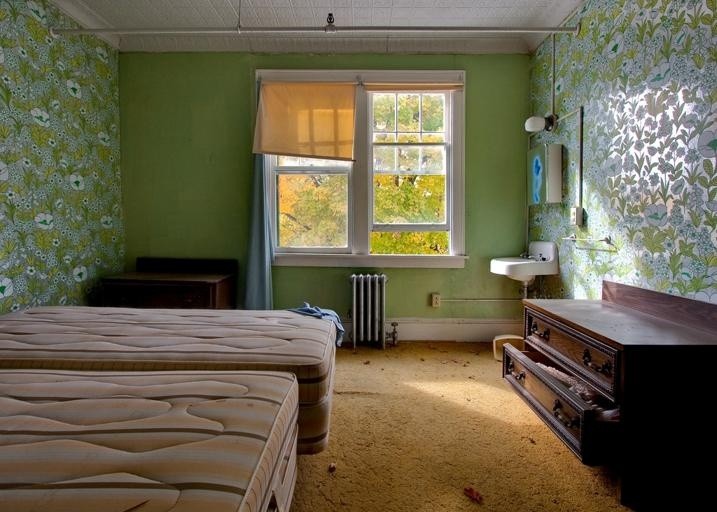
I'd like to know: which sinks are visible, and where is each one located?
[489,240,559,275]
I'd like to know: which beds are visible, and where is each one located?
[1,370,299,512]
[0,305,336,454]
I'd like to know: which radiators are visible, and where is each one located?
[350,273,386,348]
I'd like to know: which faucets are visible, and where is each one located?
[528,254,543,261]
[520,249,527,257]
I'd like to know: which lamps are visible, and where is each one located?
[524,113,557,133]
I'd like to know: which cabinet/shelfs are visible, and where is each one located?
[501,280,717,511]
[98,256,241,310]
